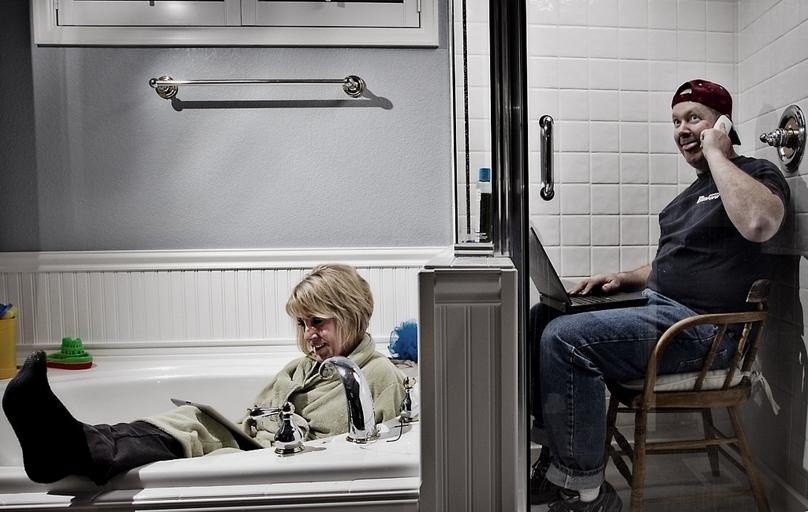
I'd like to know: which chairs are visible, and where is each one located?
[605,266,784,512]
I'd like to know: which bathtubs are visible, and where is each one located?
[0,347,420,491]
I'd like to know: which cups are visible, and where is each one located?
[0,319,18,381]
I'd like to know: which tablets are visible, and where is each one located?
[170,397,266,449]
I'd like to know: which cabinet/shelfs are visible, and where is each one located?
[31,0,446,48]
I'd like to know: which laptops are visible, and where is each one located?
[530,225,650,314]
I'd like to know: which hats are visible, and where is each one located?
[671,78,742,145]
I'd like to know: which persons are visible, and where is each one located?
[530,80,790,512]
[2,264,408,484]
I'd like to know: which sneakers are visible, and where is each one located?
[531,462,623,512]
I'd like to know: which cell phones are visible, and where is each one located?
[713,114,733,136]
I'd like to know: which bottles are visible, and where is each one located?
[474,166,492,245]
